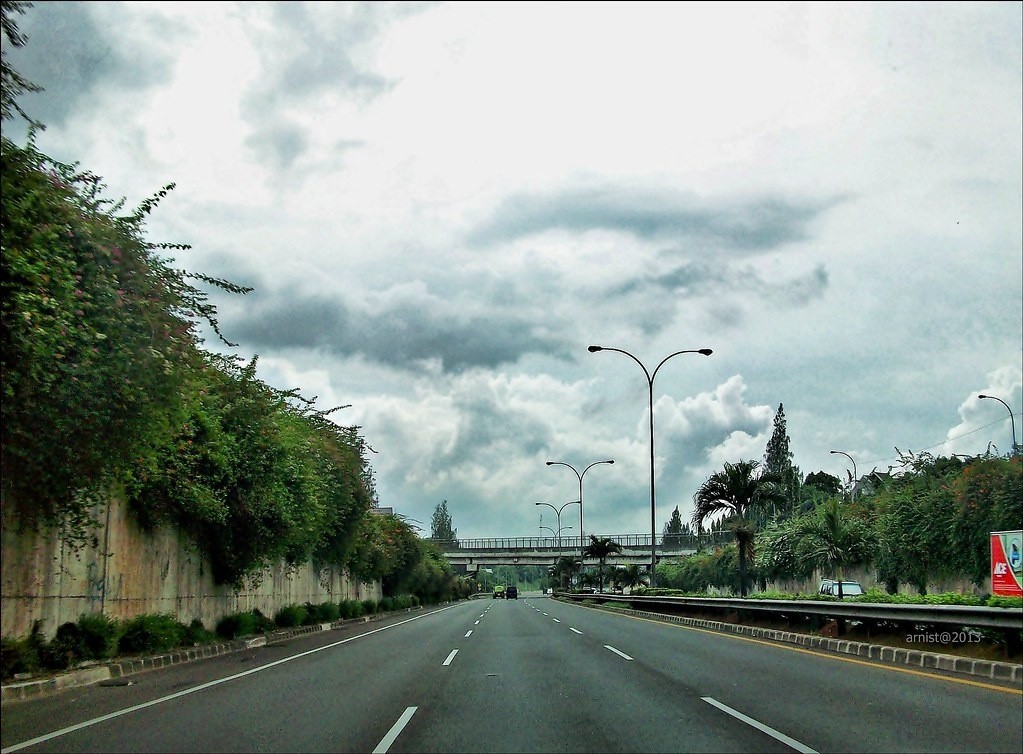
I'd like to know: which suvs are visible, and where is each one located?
[819,578,866,599]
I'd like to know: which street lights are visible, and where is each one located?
[546,459,615,570]
[588,345,713,590]
[829,450,857,488]
[979,393,1017,455]
[536,501,582,563]
[539,526,572,548]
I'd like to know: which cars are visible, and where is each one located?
[506,587,517,600]
[493,586,504,599]
[543,588,548,595]
